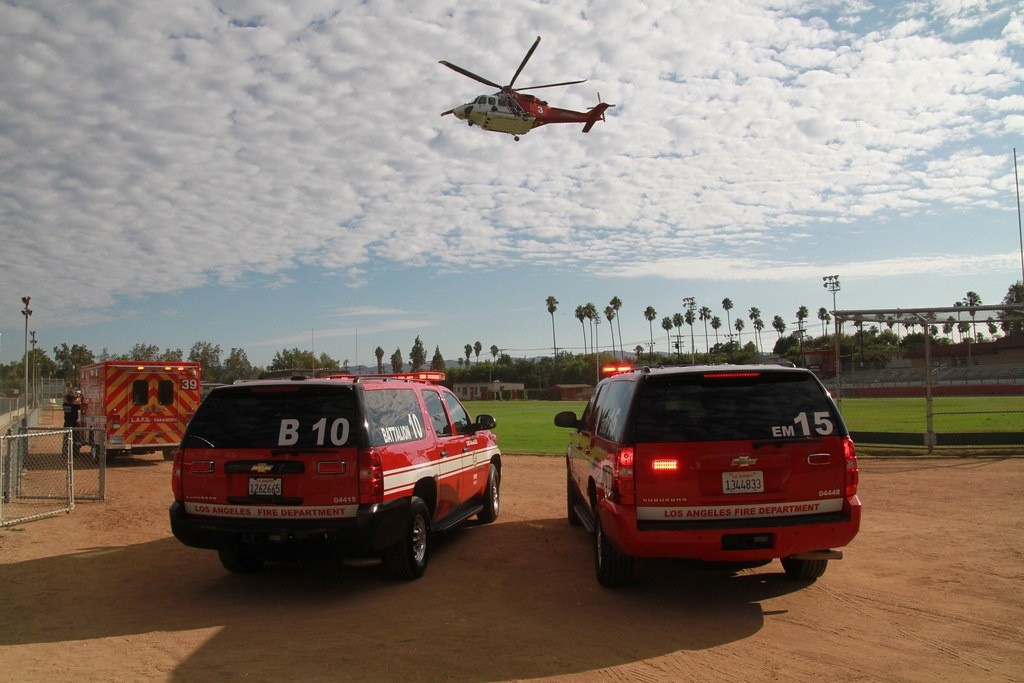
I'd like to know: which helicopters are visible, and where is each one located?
[438,35,617,136]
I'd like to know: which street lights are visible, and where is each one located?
[823,272,841,416]
[29,329,38,408]
[20,295,32,418]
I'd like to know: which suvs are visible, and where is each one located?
[553,361,862,591]
[169,371,503,584]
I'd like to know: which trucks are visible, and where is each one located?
[70,360,203,466]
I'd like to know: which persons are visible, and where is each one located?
[62,394,81,459]
